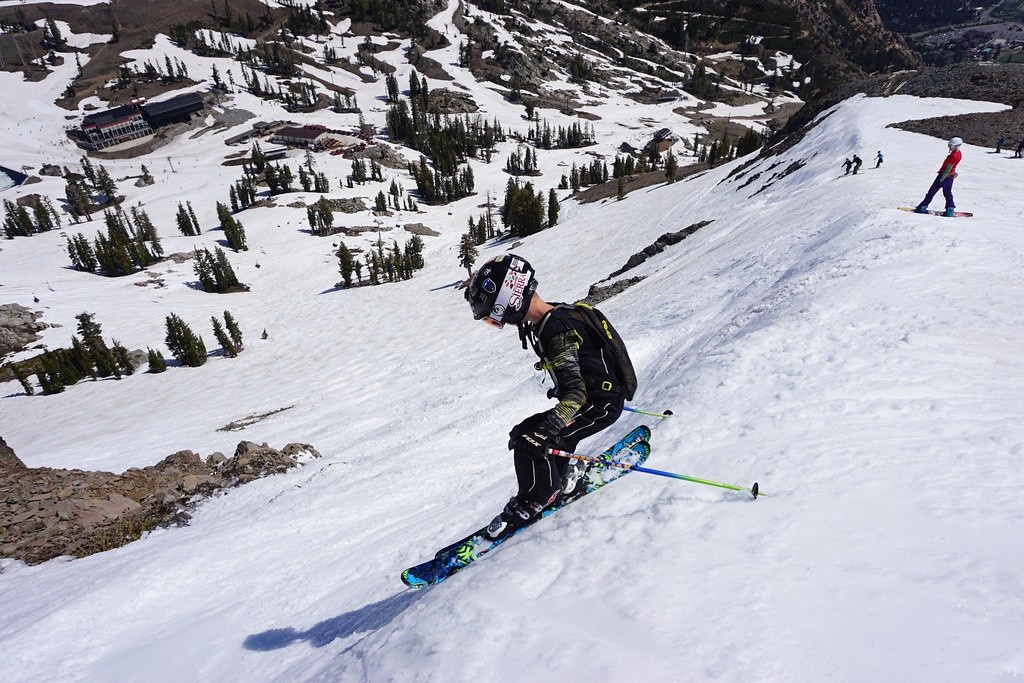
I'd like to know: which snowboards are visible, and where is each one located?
[896,206,974,218]
[1003,154,1024,160]
[985,151,1007,155]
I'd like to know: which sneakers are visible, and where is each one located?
[942,209,953,215]
[914,206,925,212]
[502,485,566,522]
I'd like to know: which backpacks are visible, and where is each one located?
[538,301,637,403]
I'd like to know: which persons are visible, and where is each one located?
[467,253,624,540]
[995,135,1006,153]
[874,151,883,168]
[842,155,862,175]
[915,137,962,217]
[1013,137,1024,158]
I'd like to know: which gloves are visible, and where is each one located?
[510,409,560,455]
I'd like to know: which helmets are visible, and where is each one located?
[948,137,963,147]
[464,255,539,332]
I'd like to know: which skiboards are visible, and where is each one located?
[835,165,887,180]
[400,424,652,590]
